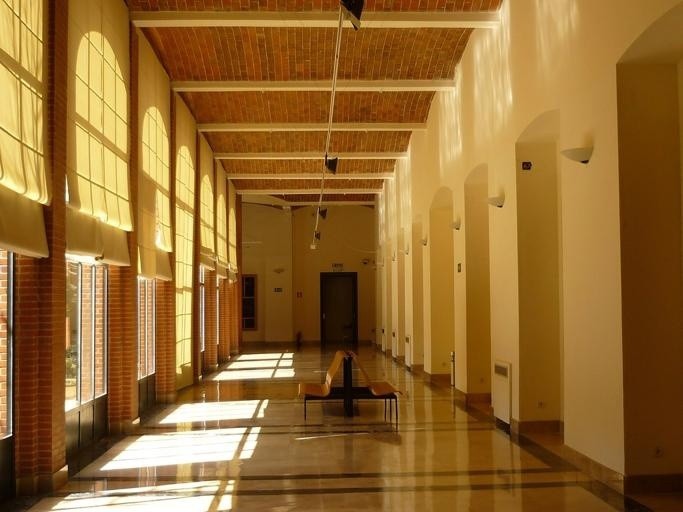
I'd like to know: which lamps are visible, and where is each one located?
[386,137,596,267]
[305,0,364,240]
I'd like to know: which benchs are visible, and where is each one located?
[296,349,346,421]
[346,348,404,433]
